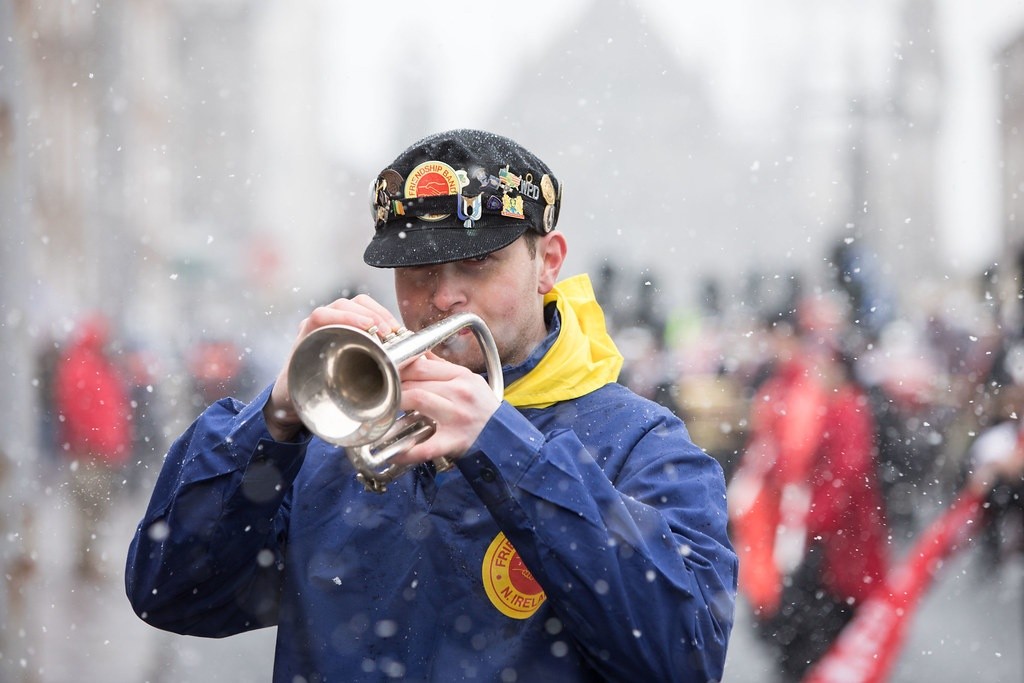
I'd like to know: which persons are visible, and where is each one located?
[594,245,1024,682]
[125,130,739,682]
[1,311,256,614]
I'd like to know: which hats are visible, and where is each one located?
[363,129,561,267]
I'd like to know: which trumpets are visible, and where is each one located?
[286,312,505,498]
